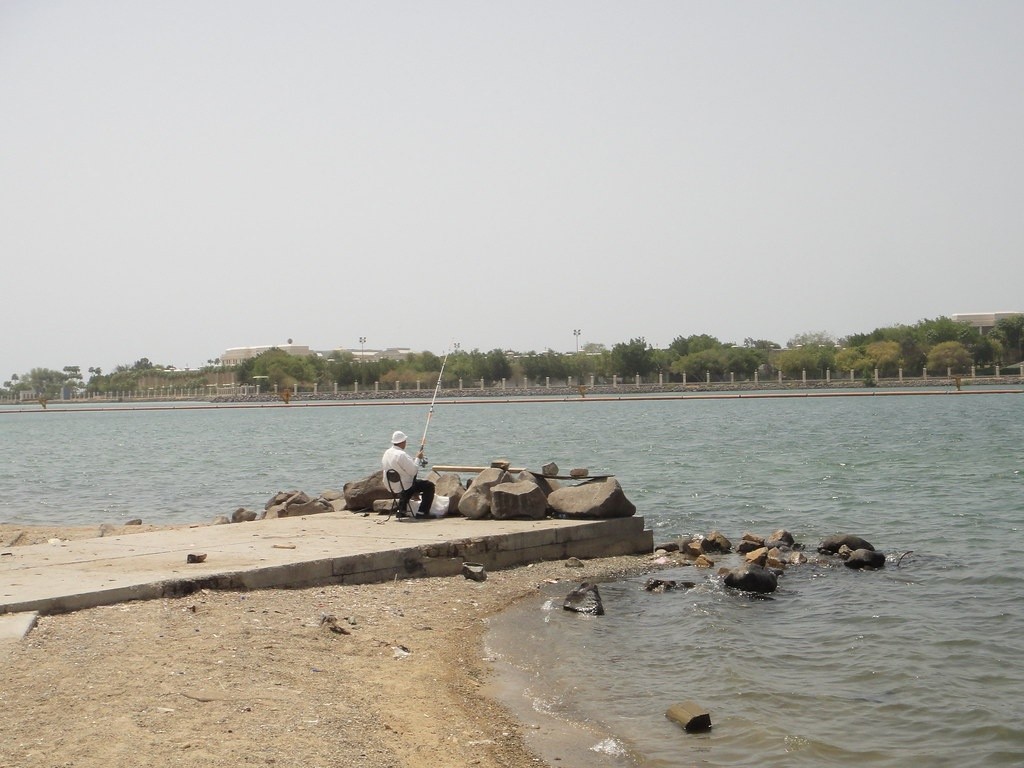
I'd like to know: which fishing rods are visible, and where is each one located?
[416,335,456,467]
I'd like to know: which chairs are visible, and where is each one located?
[385,468,416,523]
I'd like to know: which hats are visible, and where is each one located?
[391,431,408,444]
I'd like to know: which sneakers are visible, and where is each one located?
[416,512,436,519]
[396,512,410,518]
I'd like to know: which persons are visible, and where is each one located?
[382,430,437,519]
